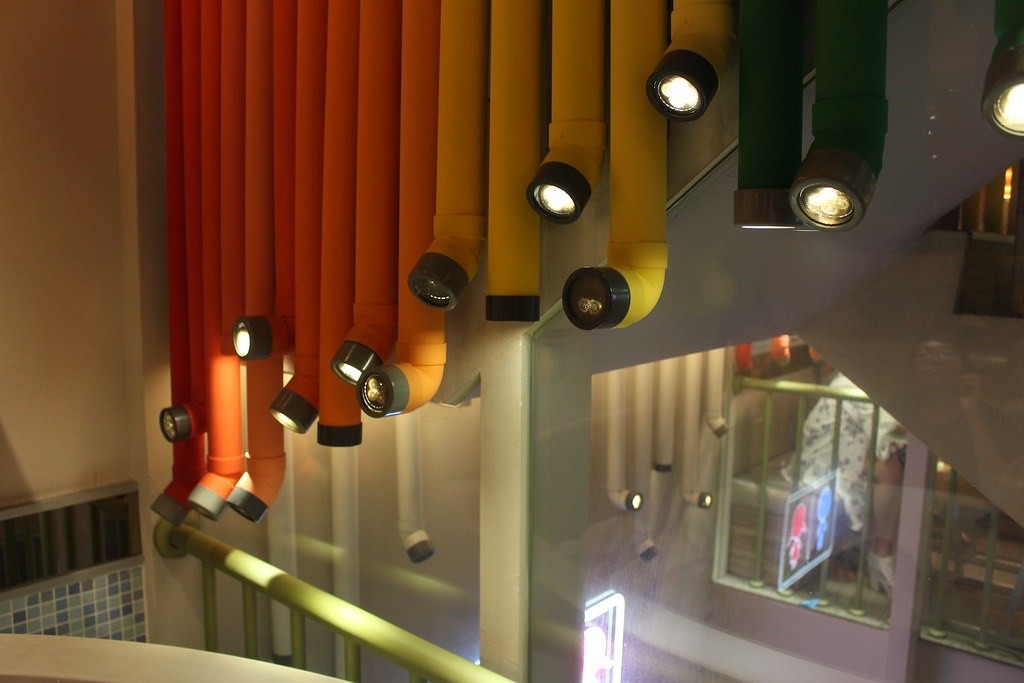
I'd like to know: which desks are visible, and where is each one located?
[934,460,1024,594]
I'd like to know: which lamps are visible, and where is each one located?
[149,0,1024,562]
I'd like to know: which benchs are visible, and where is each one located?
[733,368,841,587]
